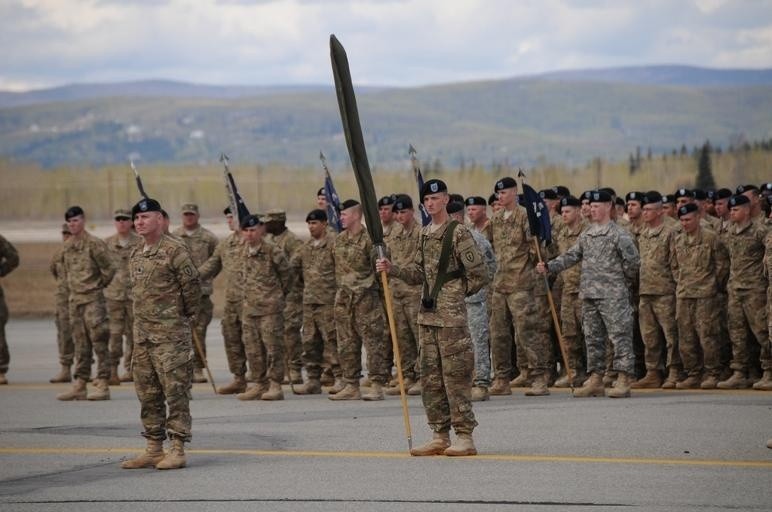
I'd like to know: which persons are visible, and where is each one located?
[120,198,202,468]
[171,203,223,383]
[51,205,188,395]
[374,180,490,456]
[197,177,772,396]
[0,235,20,384]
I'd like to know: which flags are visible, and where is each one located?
[411,158,432,226]
[225,172,249,229]
[330,33,387,246]
[324,167,343,233]
[522,183,555,254]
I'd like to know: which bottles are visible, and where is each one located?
[63,206,85,222]
[221,204,235,216]
[494,177,518,193]
[678,203,699,220]
[336,199,360,215]
[661,183,772,206]
[316,186,328,196]
[305,209,328,224]
[180,203,201,216]
[487,191,499,206]
[112,208,133,220]
[60,221,72,236]
[240,208,287,232]
[515,184,583,211]
[376,192,415,213]
[576,186,663,209]
[445,192,487,214]
[131,197,163,222]
[419,177,448,205]
[726,194,752,211]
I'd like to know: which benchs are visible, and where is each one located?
[330,33,387,246]
[225,172,249,229]
[411,158,432,226]
[324,167,343,233]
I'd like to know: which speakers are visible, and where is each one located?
[661,366,772,392]
[443,431,479,459]
[408,429,451,458]
[487,374,514,396]
[119,437,165,471]
[155,439,188,472]
[233,379,285,401]
[554,369,637,390]
[285,369,324,395]
[607,371,632,399]
[468,383,491,403]
[108,365,134,386]
[48,363,73,383]
[55,377,112,402]
[510,372,551,397]
[318,372,421,402]
[217,373,248,395]
[631,368,662,390]
[573,370,606,398]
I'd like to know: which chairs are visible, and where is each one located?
[191,367,208,384]
[0,372,10,385]
